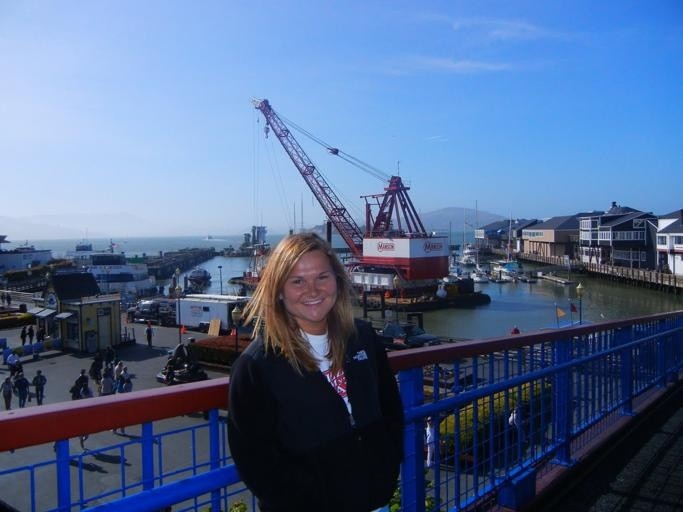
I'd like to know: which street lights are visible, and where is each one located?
[231,306,242,356]
[576,281,585,324]
[175,267,180,345]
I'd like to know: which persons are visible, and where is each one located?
[0,323,132,455]
[143,321,154,347]
[5,294,11,305]
[502,411,524,468]
[424,417,435,468]
[0,292,5,304]
[510,324,521,335]
[226,227,406,512]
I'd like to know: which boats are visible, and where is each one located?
[187,267,212,286]
[449,200,525,283]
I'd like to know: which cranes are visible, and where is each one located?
[250,97,449,298]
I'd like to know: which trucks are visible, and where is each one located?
[126,296,177,327]
